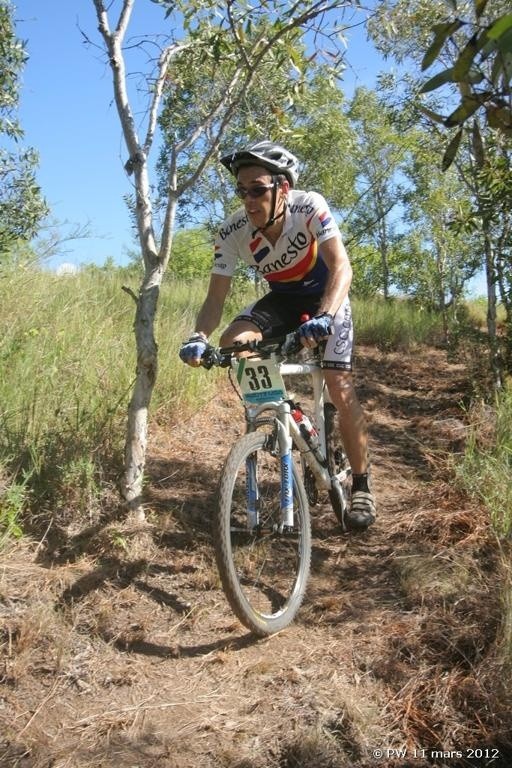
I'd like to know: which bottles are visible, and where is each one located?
[290,408,318,442]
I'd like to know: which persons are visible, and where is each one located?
[178,141,377,532]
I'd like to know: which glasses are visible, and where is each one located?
[238,186,280,198]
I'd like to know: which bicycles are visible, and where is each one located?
[198,325,374,638]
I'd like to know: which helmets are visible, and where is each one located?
[219,140,300,189]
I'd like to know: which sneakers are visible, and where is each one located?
[344,491,376,529]
